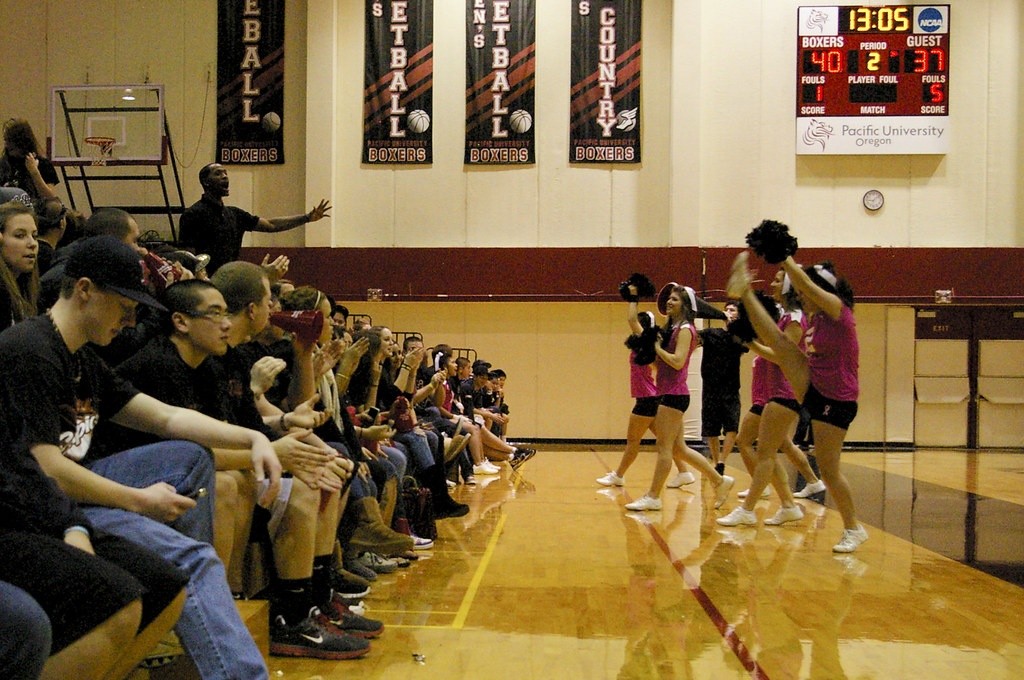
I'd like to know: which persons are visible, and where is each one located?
[596,237,869,553]
[0,117,535,680]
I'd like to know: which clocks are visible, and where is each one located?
[864,189,884,211]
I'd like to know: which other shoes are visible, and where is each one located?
[378,550,418,568]
[356,551,399,574]
[343,549,378,582]
[395,518,434,549]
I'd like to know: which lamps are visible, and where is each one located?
[123,88,135,101]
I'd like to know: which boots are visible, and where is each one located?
[419,464,470,520]
[349,497,414,553]
[313,540,370,599]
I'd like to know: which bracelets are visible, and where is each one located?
[400,362,412,371]
[368,383,378,387]
[360,426,365,438]
[63,526,90,537]
[280,413,289,431]
[303,214,311,223]
[336,371,352,382]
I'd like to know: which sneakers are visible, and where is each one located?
[667,488,695,503]
[711,475,734,509]
[727,251,752,300]
[832,524,869,553]
[738,499,771,510]
[737,485,770,498]
[763,504,804,526]
[793,498,828,518]
[596,471,624,487]
[793,479,826,498]
[270,606,370,659]
[321,589,385,637]
[833,555,869,576]
[666,471,695,489]
[473,457,500,474]
[625,512,662,526]
[624,494,662,511]
[715,508,757,527]
[764,526,804,546]
[596,488,625,501]
[508,447,536,470]
[715,528,757,546]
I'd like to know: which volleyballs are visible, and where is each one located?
[510,109,532,134]
[407,109,431,133]
[617,109,637,133]
[262,111,281,133]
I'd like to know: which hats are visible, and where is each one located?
[158,251,210,274]
[64,235,169,312]
[472,359,492,377]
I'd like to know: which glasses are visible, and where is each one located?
[181,307,233,322]
